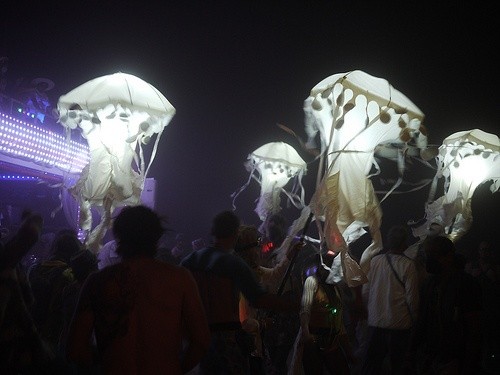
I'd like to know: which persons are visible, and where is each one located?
[1,197,498,374]
